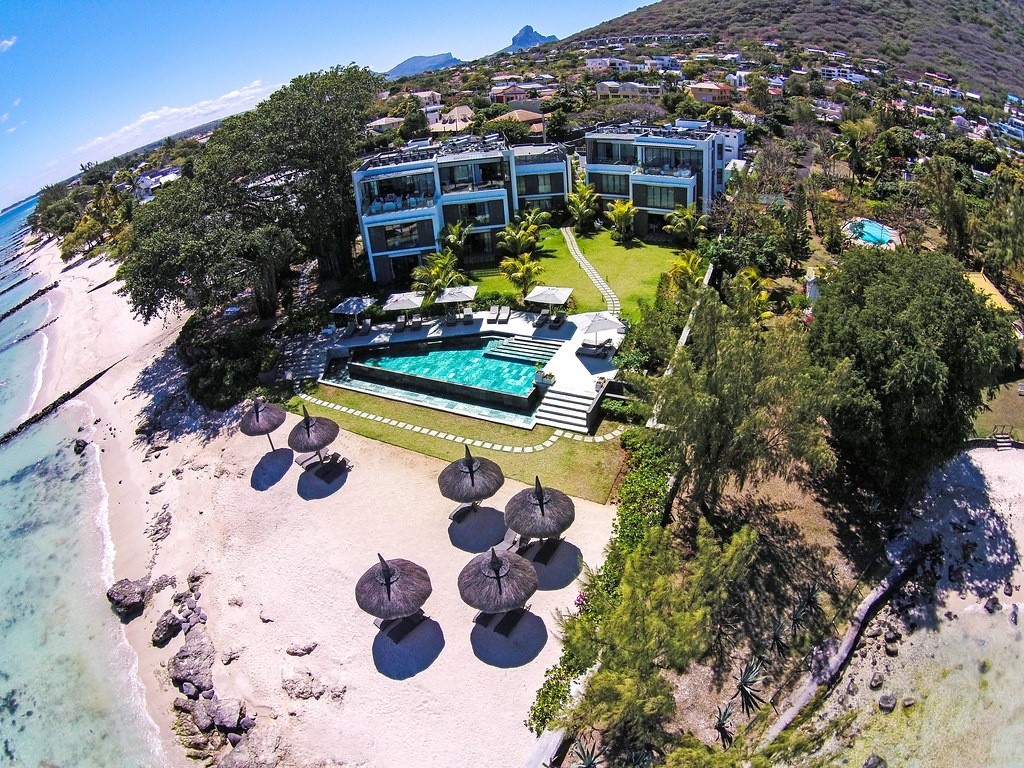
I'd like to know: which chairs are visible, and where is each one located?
[575,346,605,359]
[373,617,396,631]
[387,608,428,644]
[302,447,327,471]
[446,310,457,326]
[410,313,422,329]
[498,305,511,324]
[315,451,340,481]
[342,320,355,337]
[358,318,372,336]
[321,457,351,484]
[533,309,550,328]
[533,535,562,566]
[487,305,499,323]
[473,611,495,628]
[494,607,528,637]
[295,445,323,466]
[582,338,612,349]
[395,315,406,331]
[449,499,481,524]
[549,312,567,329]
[462,308,474,324]
[493,527,517,552]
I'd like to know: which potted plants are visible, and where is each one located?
[597,376,605,384]
[543,373,555,385]
[536,360,545,383]
[594,381,603,391]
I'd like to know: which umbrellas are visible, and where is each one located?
[288,403,340,468]
[383,291,427,322]
[572,310,624,345]
[434,285,478,319]
[354,553,433,621]
[437,445,505,511]
[329,296,380,326]
[503,474,576,546]
[458,546,539,614]
[238,395,286,452]
[523,286,575,315]
